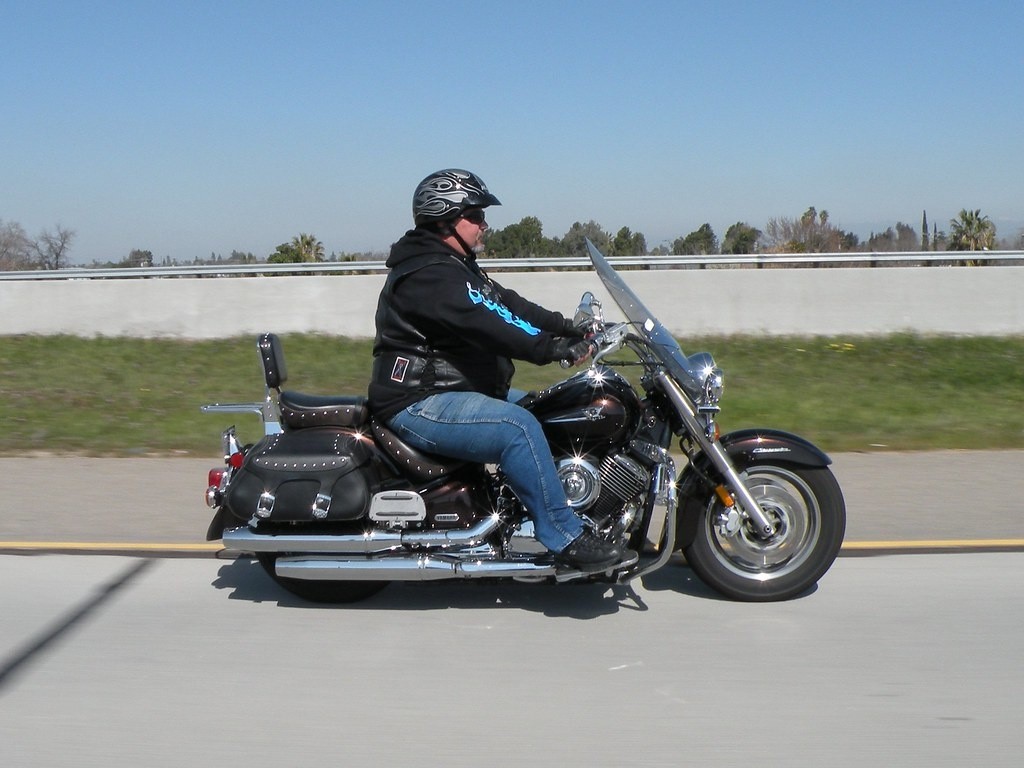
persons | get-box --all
[367,168,640,572]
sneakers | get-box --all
[553,530,622,570]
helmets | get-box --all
[412,168,501,231]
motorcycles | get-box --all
[198,235,848,603]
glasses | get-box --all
[459,209,485,224]
